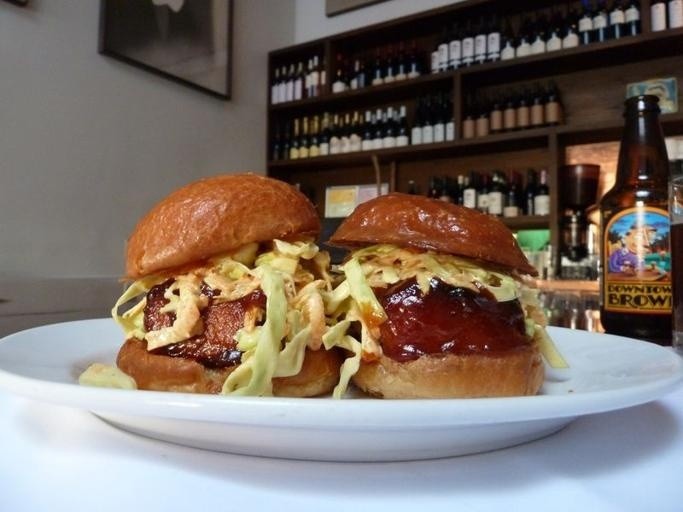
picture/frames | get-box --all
[96,0,233,101]
[325,0,386,17]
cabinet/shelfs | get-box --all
[264,0,682,346]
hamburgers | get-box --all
[323,192,569,398]
[100,171,332,395]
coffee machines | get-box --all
[558,161,604,280]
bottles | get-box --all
[601,94,681,342]
[409,95,457,146]
[503,0,682,76]
[268,55,329,105]
[403,168,550,218]
[272,110,365,160]
[429,20,502,76]
[333,37,424,96]
[462,79,566,142]
[364,105,408,153]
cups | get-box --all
[666,173,683,345]
